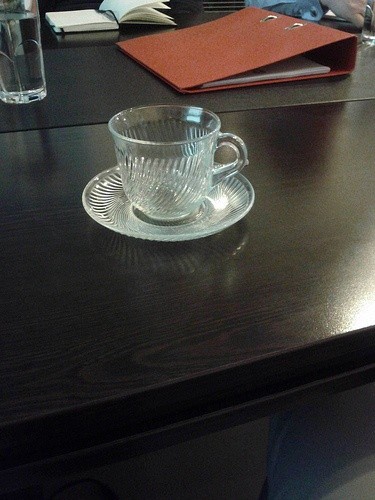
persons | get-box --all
[245,0,375,30]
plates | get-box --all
[81,164,253,241]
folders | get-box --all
[114,6,359,95]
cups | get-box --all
[107,103,248,221]
[0,0,48,105]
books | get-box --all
[44,0,178,34]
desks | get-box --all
[1,13,375,499]
